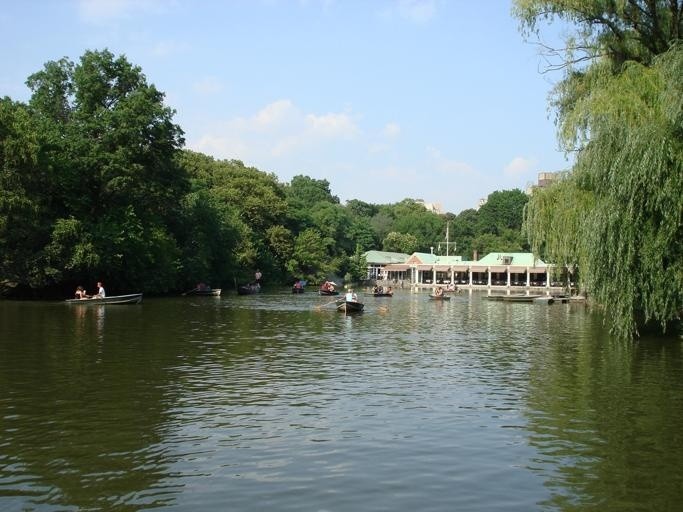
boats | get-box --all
[333,301,365,315]
[483,292,541,303]
[63,293,146,305]
[289,287,306,294]
[178,287,221,297]
[428,293,450,302]
[316,289,340,297]
[238,282,261,294]
[368,291,394,298]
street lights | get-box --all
[437,241,457,255]
[432,256,440,284]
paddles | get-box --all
[181,288,197,295]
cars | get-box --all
[425,278,576,287]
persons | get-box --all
[343,277,457,304]
[196,268,335,293]
[75,282,105,299]
